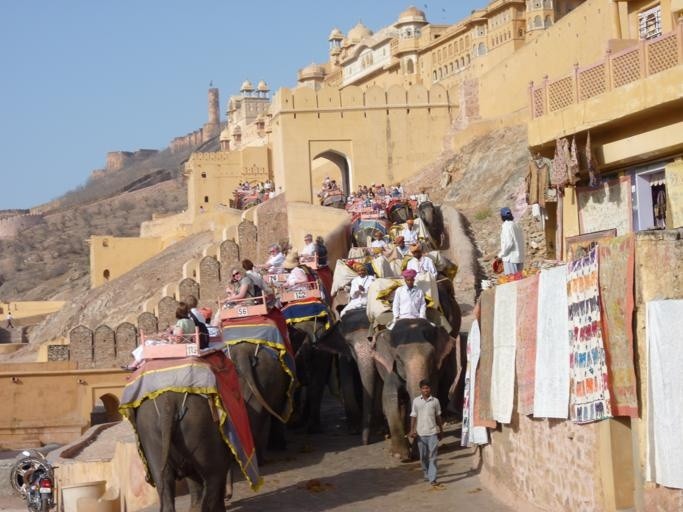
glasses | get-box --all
[233,272,238,277]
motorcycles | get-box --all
[20,462,59,511]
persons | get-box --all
[184,294,205,328]
[5,311,13,328]
[495,204,524,273]
[218,175,448,322]
[199,306,213,324]
[120,302,194,371]
[410,380,444,485]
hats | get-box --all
[407,220,414,224]
[396,235,404,242]
[374,231,383,236]
[282,256,300,269]
[402,269,416,278]
[409,244,421,252]
[500,207,512,217]
[198,306,212,318]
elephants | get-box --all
[322,194,345,209]
[369,317,459,462]
[222,342,289,468]
[313,307,378,447]
[133,390,236,512]
[286,319,332,435]
[388,202,414,223]
[436,274,462,338]
[417,200,446,249]
[355,221,387,246]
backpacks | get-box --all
[189,311,209,349]
[247,277,262,304]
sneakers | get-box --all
[424,477,437,486]
